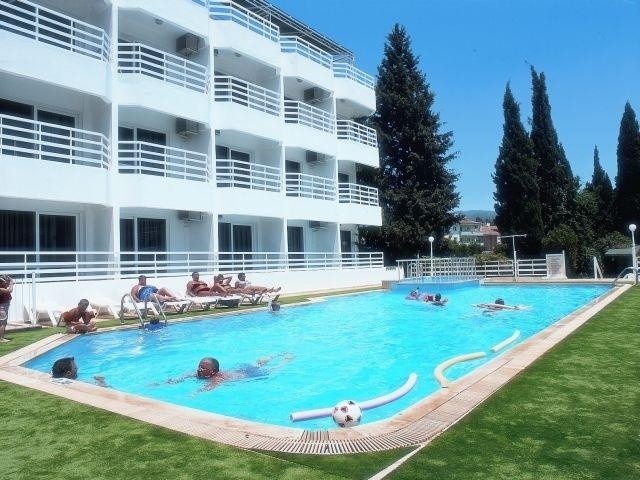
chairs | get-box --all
[24,284,281,328]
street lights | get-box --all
[428,236,435,277]
[629,224,637,280]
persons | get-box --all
[142,318,168,330]
[0,274,14,344]
[470,296,530,317]
[130,276,181,304]
[63,298,97,334]
[404,286,448,307]
[235,273,281,293]
[51,356,107,388]
[213,274,267,297]
[186,272,233,297]
[144,351,294,403]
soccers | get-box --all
[333,400,361,427]
